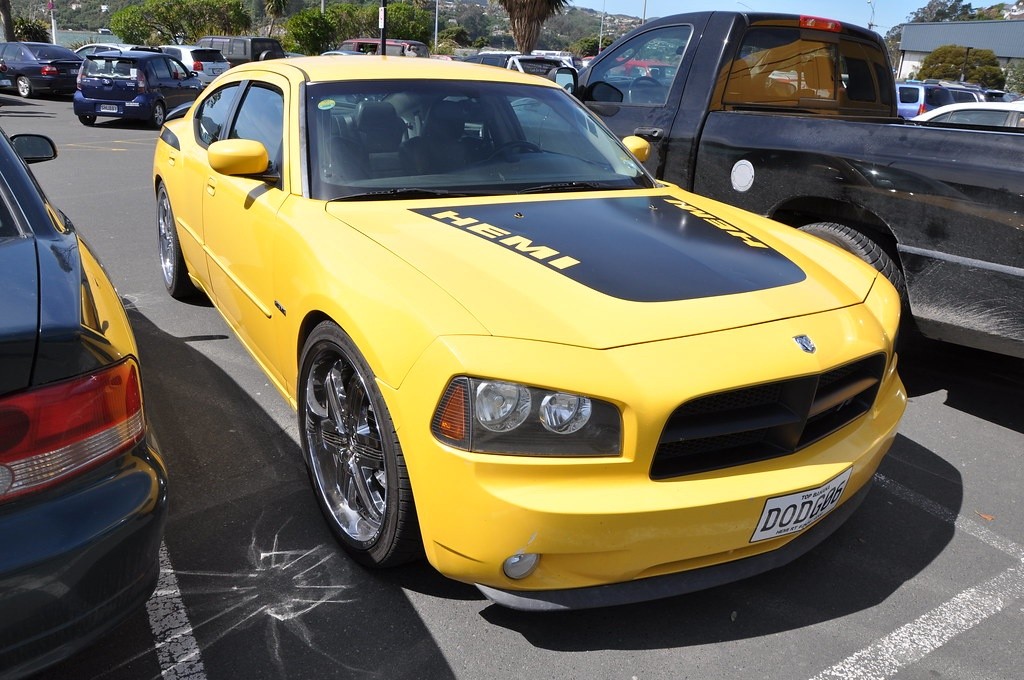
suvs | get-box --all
[192,35,286,66]
[460,54,579,77]
[335,39,430,58]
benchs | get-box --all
[328,101,403,182]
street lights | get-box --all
[867,0,876,31]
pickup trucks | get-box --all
[547,11,1022,360]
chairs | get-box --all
[400,99,478,178]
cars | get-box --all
[478,49,677,85]
[896,76,1024,105]
[157,44,232,87]
[895,81,956,121]
[321,51,364,55]
[152,54,911,615]
[1,42,85,100]
[74,43,163,59]
[430,54,461,62]
[909,100,1024,128]
[72,50,204,128]
[1,128,171,680]
[284,51,306,58]
